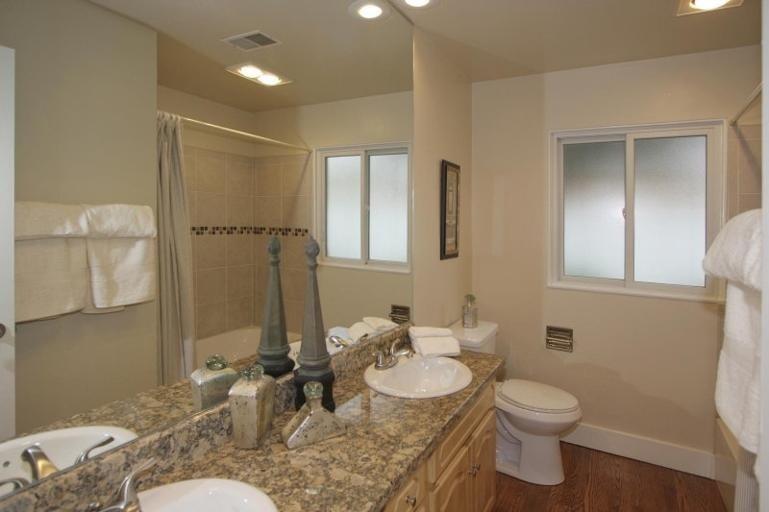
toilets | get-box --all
[449,318,585,487]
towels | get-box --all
[14,201,159,325]
[703,206,765,456]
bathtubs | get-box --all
[195,325,302,368]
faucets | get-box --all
[328,336,350,348]
[20,444,60,482]
[84,499,139,512]
[390,337,414,361]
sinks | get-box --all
[0,425,138,495]
[364,354,474,399]
[287,337,346,370]
[136,478,279,512]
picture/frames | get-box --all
[441,159,459,260]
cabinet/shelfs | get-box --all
[370,378,499,510]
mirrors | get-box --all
[0,1,419,497]
